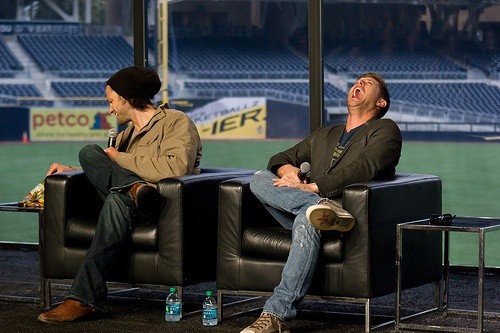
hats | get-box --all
[105,66,161,104]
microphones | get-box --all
[298,161,311,182]
[108,127,118,147]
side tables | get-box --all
[393,215,500,333]
[0,201,43,302]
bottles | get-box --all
[202,291,218,326]
[165,287,181,322]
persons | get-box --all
[38,66,203,325]
[239,74,402,333]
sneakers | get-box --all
[307,200,355,231]
[241,310,287,333]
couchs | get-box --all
[211,173,444,333]
[41,167,259,311]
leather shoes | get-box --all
[132,183,168,214]
[38,299,93,322]
